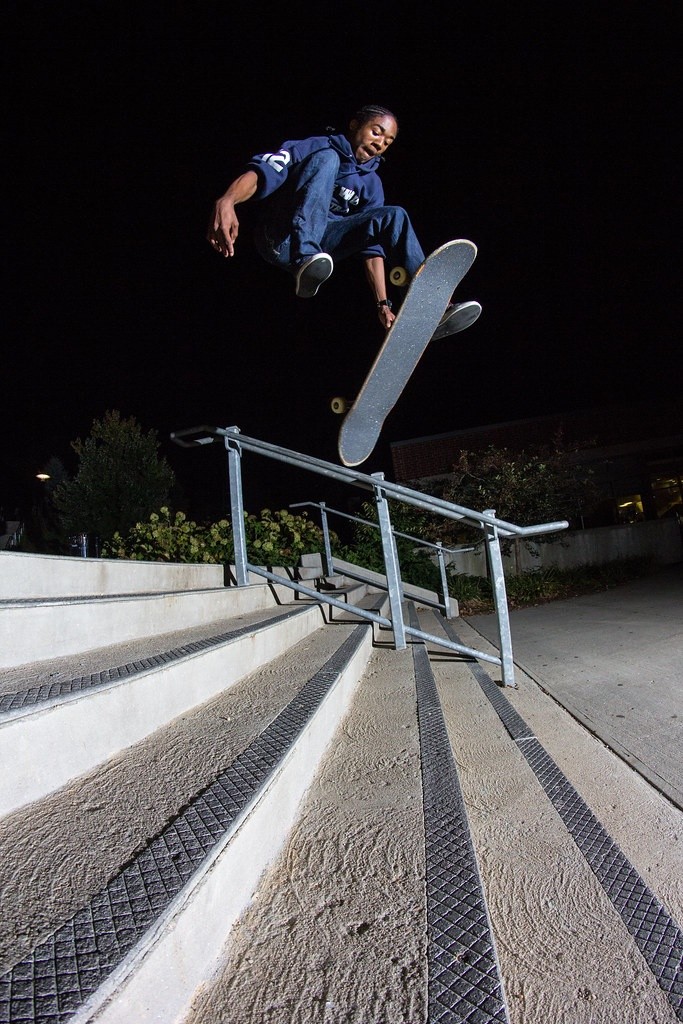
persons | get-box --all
[207,105,482,340]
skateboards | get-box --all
[329,236,479,469]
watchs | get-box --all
[376,299,393,309]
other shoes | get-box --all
[286,252,333,297]
[428,298,483,342]
